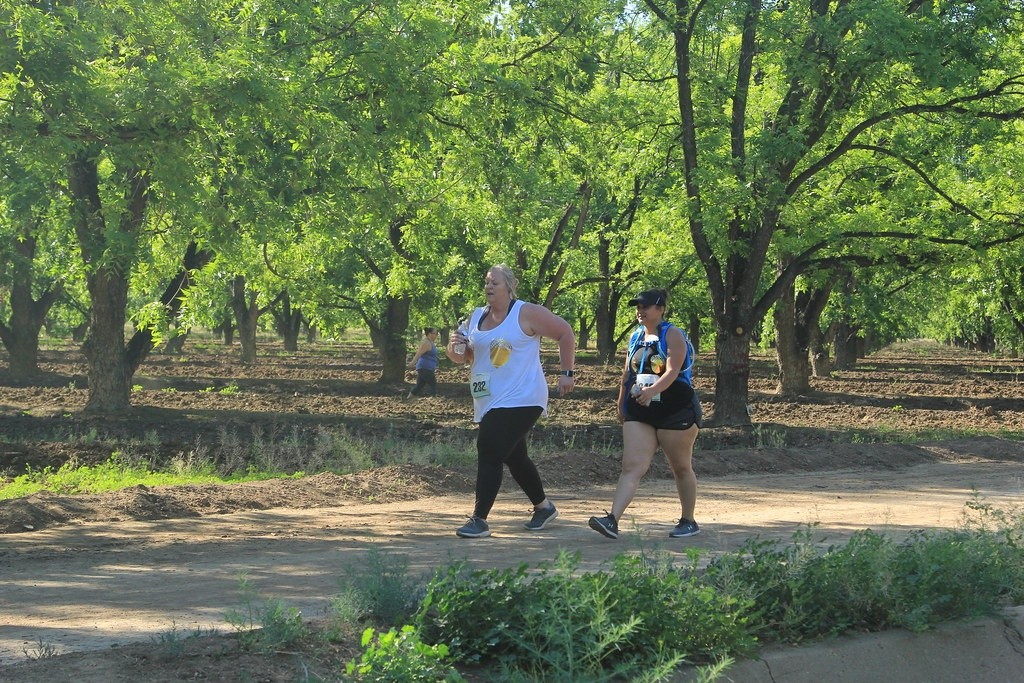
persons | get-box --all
[407,328,440,399]
[588,288,703,539]
[445,262,577,538]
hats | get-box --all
[628,292,666,306]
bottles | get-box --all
[453,321,468,354]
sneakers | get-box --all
[589,508,618,539]
[524,501,558,530]
[456,514,491,538]
[669,518,701,538]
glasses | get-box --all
[430,332,438,335]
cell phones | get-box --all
[630,384,652,407]
[453,332,466,355]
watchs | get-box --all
[562,370,573,377]
